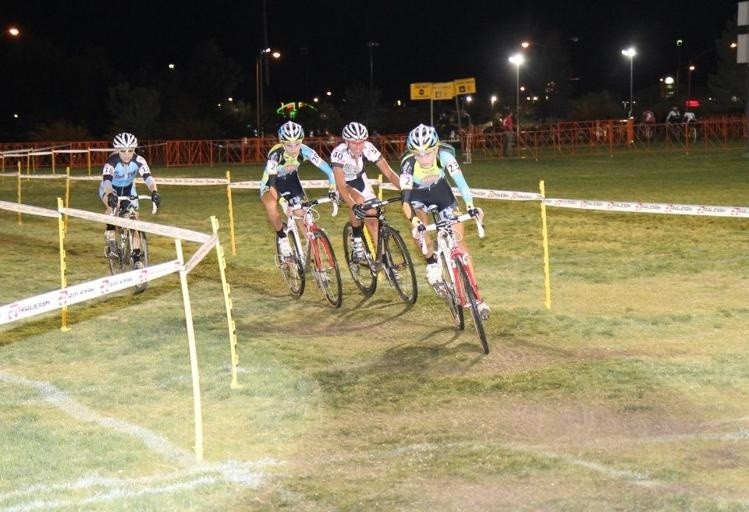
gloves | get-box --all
[283,192,300,207]
[466,206,479,217]
[108,193,118,207]
[353,204,366,220]
[412,216,427,233]
[152,190,160,205]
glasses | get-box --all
[121,150,133,152]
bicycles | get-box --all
[413,204,491,356]
[342,194,418,306]
[274,198,344,310]
[102,194,159,293]
[667,123,698,145]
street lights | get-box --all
[509,54,523,159]
[621,49,635,118]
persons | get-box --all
[97,130,163,271]
[397,123,494,321]
[259,120,338,261]
[328,120,404,258]
[637,105,697,145]
[502,111,515,158]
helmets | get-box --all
[408,124,439,153]
[342,122,368,141]
[113,133,137,148]
[278,121,304,144]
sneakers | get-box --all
[477,303,490,315]
[130,256,144,268]
[278,237,294,257]
[352,240,364,258]
[108,247,119,257]
[427,263,440,285]
